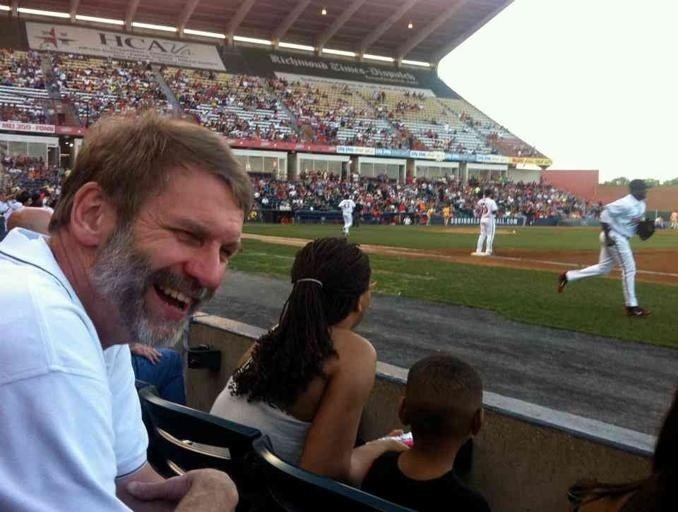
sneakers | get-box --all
[557,269,568,294]
[623,305,654,318]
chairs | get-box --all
[137,382,418,512]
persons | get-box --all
[360,349,494,512]
[472,188,498,257]
[206,234,411,488]
[556,177,655,318]
[654,214,667,229]
[126,335,191,408]
[668,208,677,232]
[1,108,260,512]
[1,155,72,236]
[562,383,676,512]
[244,168,604,227]
[2,46,540,158]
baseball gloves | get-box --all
[637,218,654,240]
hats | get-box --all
[627,179,654,191]
